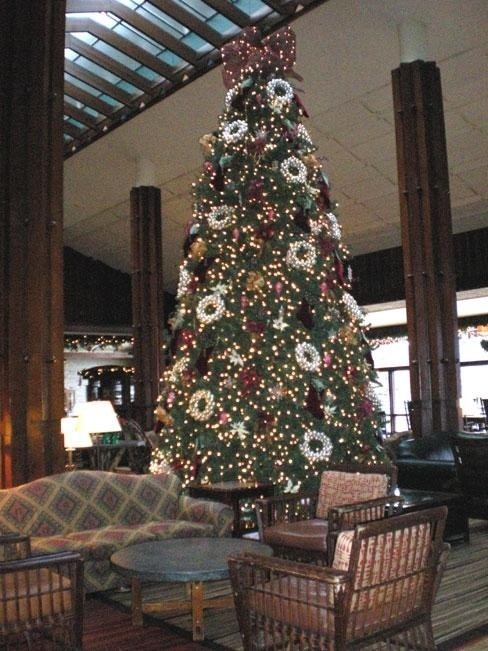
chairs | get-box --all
[227,502,453,649]
[263,463,398,566]
[449,431,488,520]
[0,531,84,651]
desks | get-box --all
[79,440,146,473]
[187,479,273,539]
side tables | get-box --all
[385,488,470,548]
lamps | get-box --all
[59,400,123,469]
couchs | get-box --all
[0,469,236,597]
[384,430,488,493]
